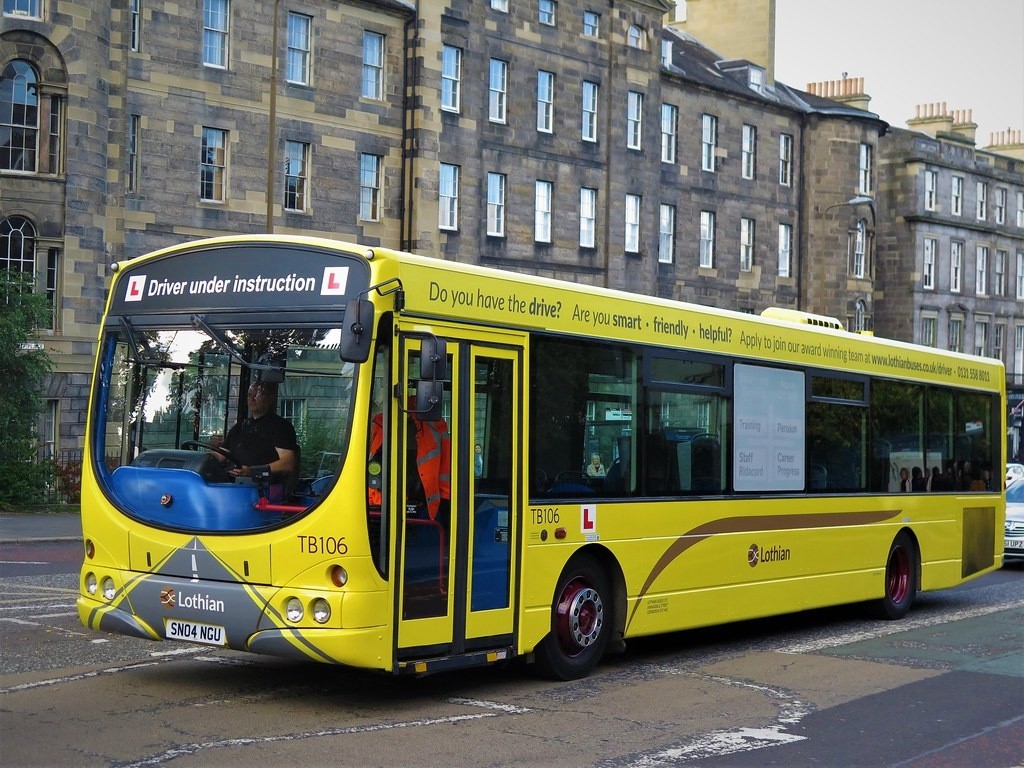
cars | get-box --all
[1003,476,1024,566]
[1004,463,1024,489]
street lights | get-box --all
[819,196,874,316]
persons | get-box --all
[205,379,300,503]
[899,454,1024,492]
[474,444,483,479]
[368,395,451,520]
[586,454,606,476]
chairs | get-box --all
[473,423,992,500]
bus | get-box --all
[77,233,1003,683]
